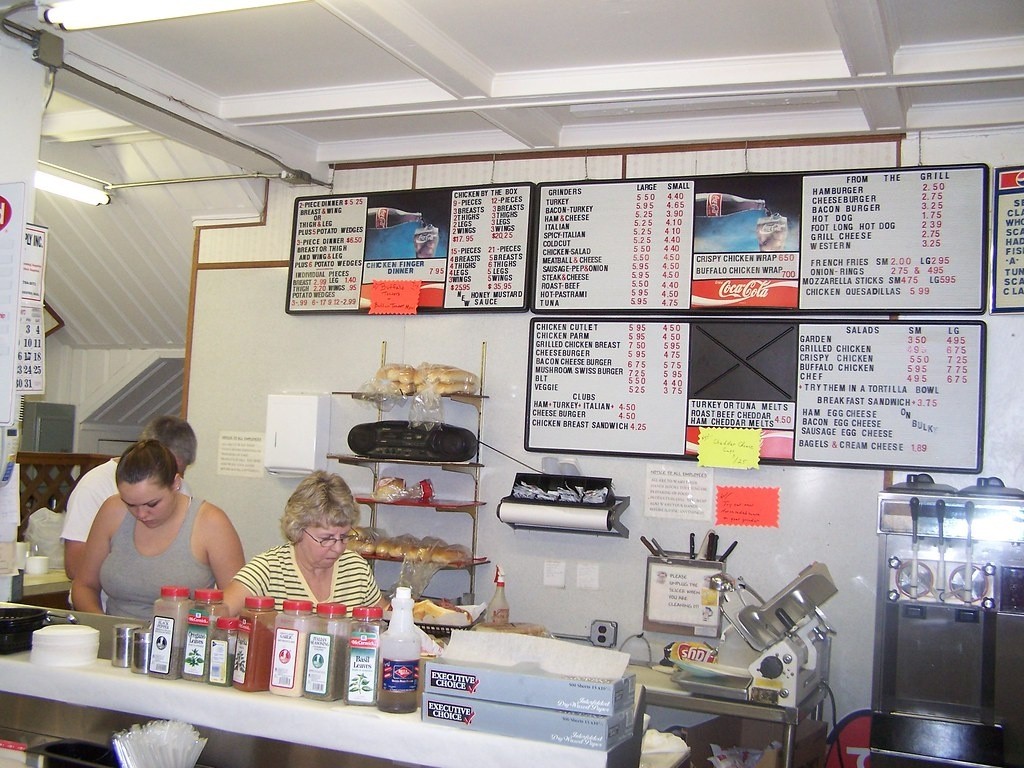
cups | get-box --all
[414,228,439,258]
[26,556,49,574]
[757,217,787,251]
[17,543,30,568]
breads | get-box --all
[373,477,406,500]
[411,598,453,621]
[384,366,478,396]
[345,527,466,563]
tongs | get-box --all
[45,610,79,624]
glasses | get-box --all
[302,526,359,547]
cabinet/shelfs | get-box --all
[325,337,488,642]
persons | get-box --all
[224,470,390,619]
[64,415,246,620]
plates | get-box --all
[31,624,100,667]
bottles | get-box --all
[378,587,419,714]
[366,207,423,229]
[696,193,765,217]
[181,589,228,683]
[131,631,152,674]
[112,624,144,667]
[303,603,350,701]
[269,600,314,697]
[486,564,510,624]
[148,586,193,680]
[344,607,387,705]
[209,618,238,687]
[232,596,277,692]
